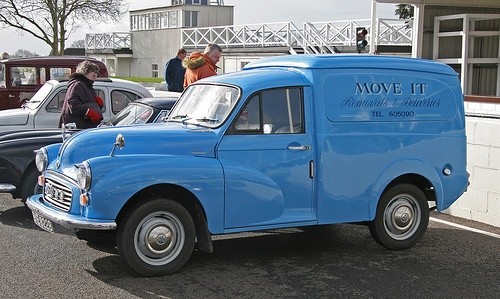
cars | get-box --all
[0,98,179,206]
[0,81,155,133]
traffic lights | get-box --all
[355,27,369,51]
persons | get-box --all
[181,43,222,89]
[164,48,186,93]
[58,60,104,129]
[1,52,21,86]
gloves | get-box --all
[84,108,101,124]
[94,95,103,109]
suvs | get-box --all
[25,53,471,277]
[0,56,111,111]
